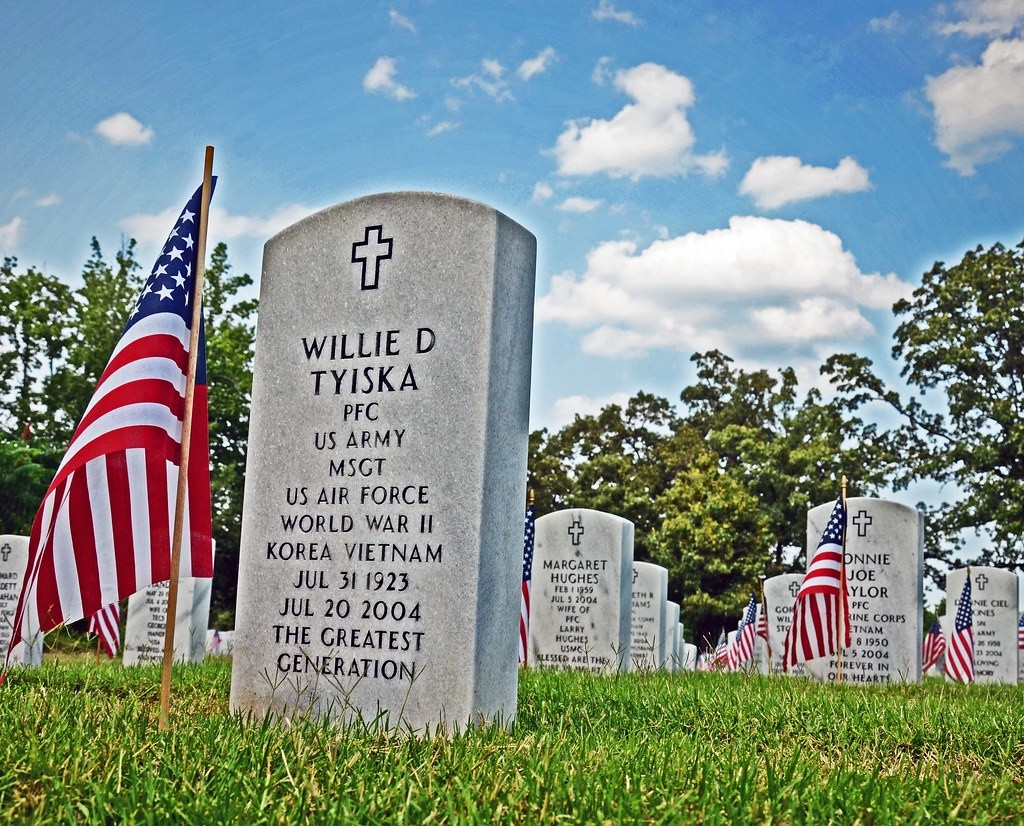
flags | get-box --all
[0,175,219,685]
[756,596,769,642]
[943,576,975,686]
[783,494,851,672]
[1018,612,1024,649]
[696,632,729,672]
[729,595,757,668]
[517,505,535,664]
[922,618,946,672]
